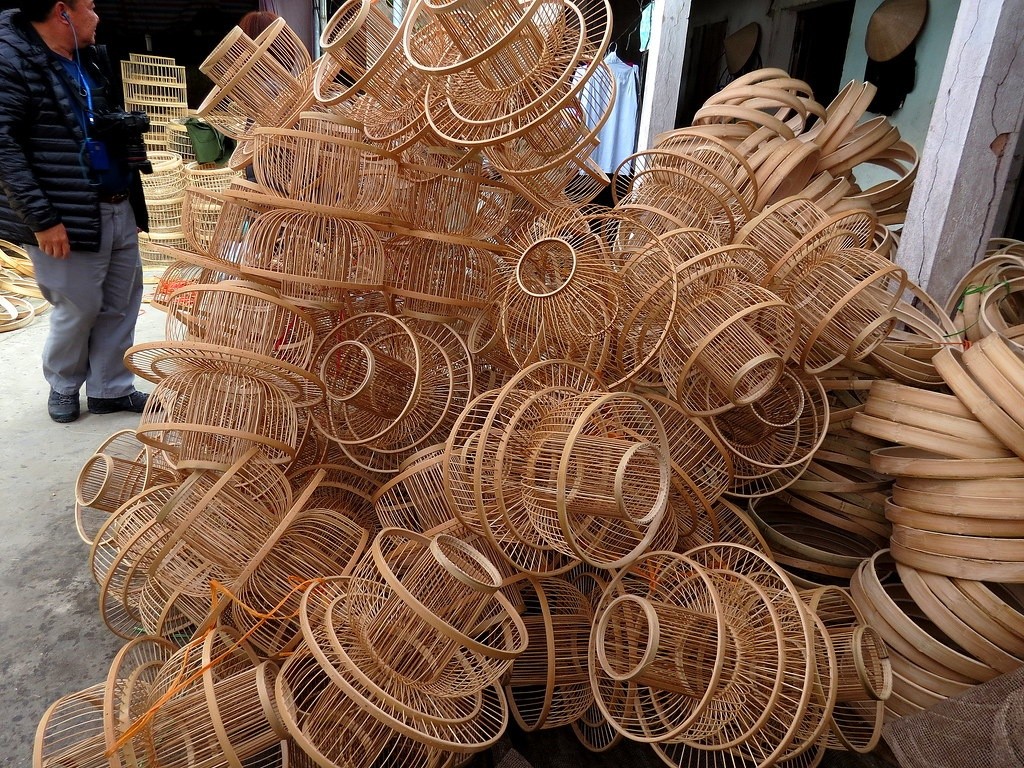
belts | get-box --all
[98,189,131,204]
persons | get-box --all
[1,0,163,423]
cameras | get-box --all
[97,106,154,174]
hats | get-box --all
[723,22,760,76]
[865,0,927,62]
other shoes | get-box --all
[47,386,80,424]
[88,391,168,414]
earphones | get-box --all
[61,11,69,19]
